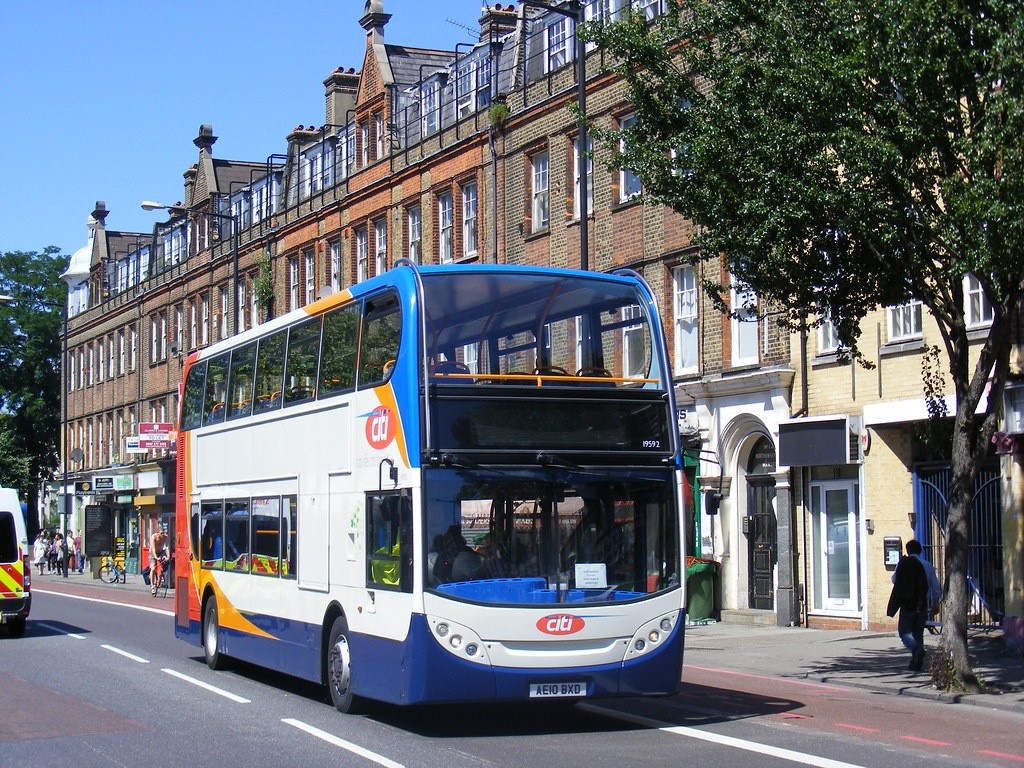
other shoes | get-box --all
[908,660,922,671]
[150,587,155,594]
[909,648,925,669]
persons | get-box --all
[148,527,171,594]
[891,540,942,671]
[73,529,84,574]
[66,531,76,571]
[34,531,47,576]
[56,534,67,575]
[48,531,57,574]
[433,505,636,591]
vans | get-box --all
[0,489,32,637]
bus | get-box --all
[174,258,723,713]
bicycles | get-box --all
[151,557,171,599]
[99,551,126,584]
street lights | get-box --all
[0,295,68,579]
[142,201,238,335]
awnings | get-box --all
[862,386,995,429]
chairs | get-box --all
[256,395,271,410]
[271,391,281,407]
[474,377,503,385]
[532,366,575,387]
[211,402,225,420]
[577,368,617,388]
[430,361,475,384]
[312,380,344,398]
[383,360,396,379]
[504,372,533,385]
[232,403,243,416]
[239,399,251,415]
[291,386,312,400]
